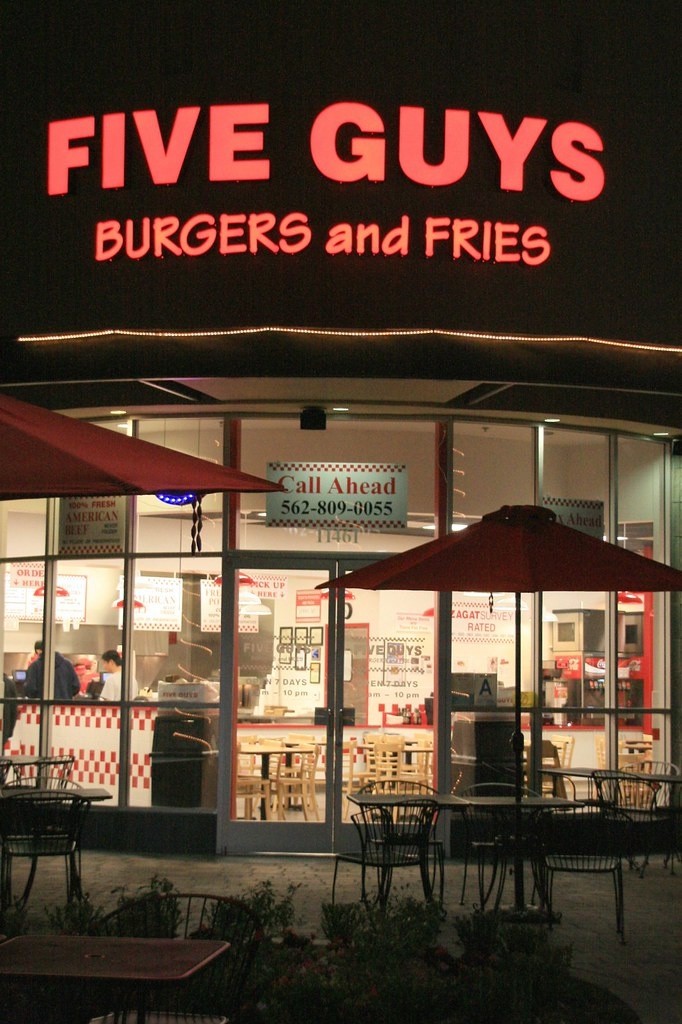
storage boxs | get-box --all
[156,681,220,717]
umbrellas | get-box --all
[0,394,288,501]
[314,503,681,907]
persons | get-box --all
[97,649,139,701]
[23,641,81,701]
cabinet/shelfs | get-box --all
[542,608,643,726]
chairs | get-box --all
[237,732,325,821]
[594,733,656,810]
[343,733,434,824]
[459,759,682,942]
[87,893,264,1024]
[523,735,576,812]
[331,779,444,913]
[0,756,92,902]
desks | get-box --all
[0,934,231,1024]
[625,737,653,771]
[537,768,682,870]
[239,742,314,820]
[356,744,434,790]
[0,755,75,788]
[0,788,114,912]
[346,794,585,923]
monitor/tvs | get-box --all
[426,698,472,725]
[314,708,355,727]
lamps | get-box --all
[421,608,435,617]
[239,592,272,616]
[113,599,144,609]
[33,585,70,598]
[321,589,355,603]
[299,405,327,430]
[337,600,338,603]
[213,572,255,587]
